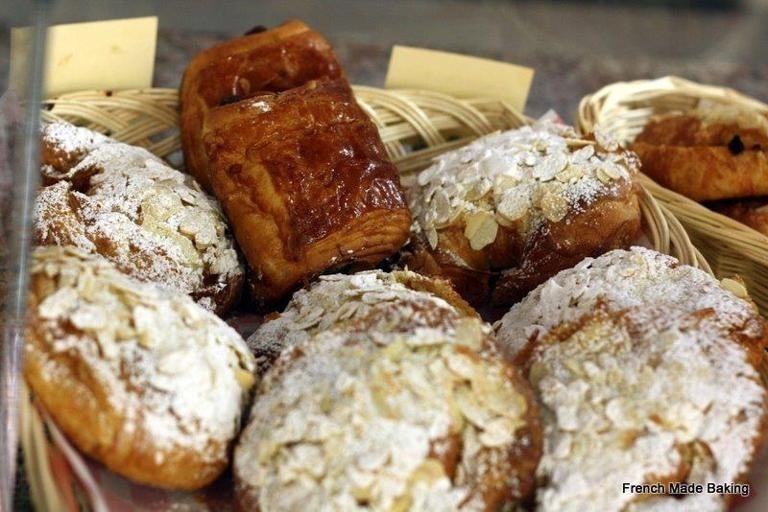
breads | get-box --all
[20,18,768,512]
